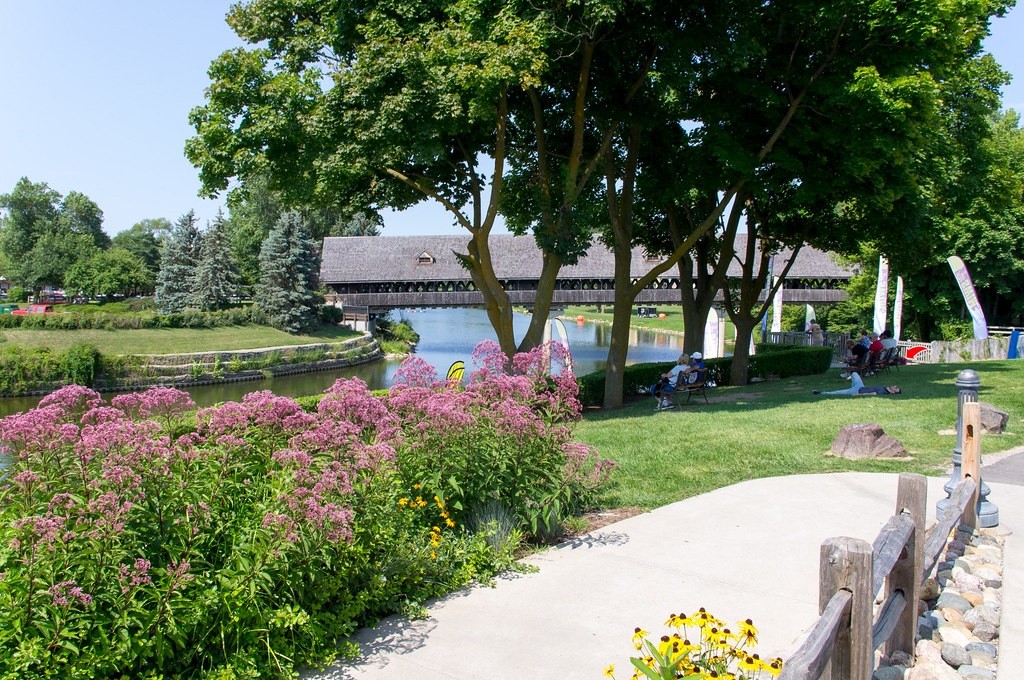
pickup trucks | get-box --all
[11,304,57,315]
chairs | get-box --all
[846,345,902,379]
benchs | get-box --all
[654,368,709,411]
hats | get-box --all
[690,352,702,359]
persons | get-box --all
[839,329,898,378]
[649,351,705,409]
[811,372,901,396]
[802,318,824,346]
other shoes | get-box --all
[870,372,874,375]
[663,405,674,408]
[864,373,870,376]
[841,373,850,378]
[653,406,665,411]
[812,390,821,395]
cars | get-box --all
[43,290,125,304]
[0,303,20,316]
